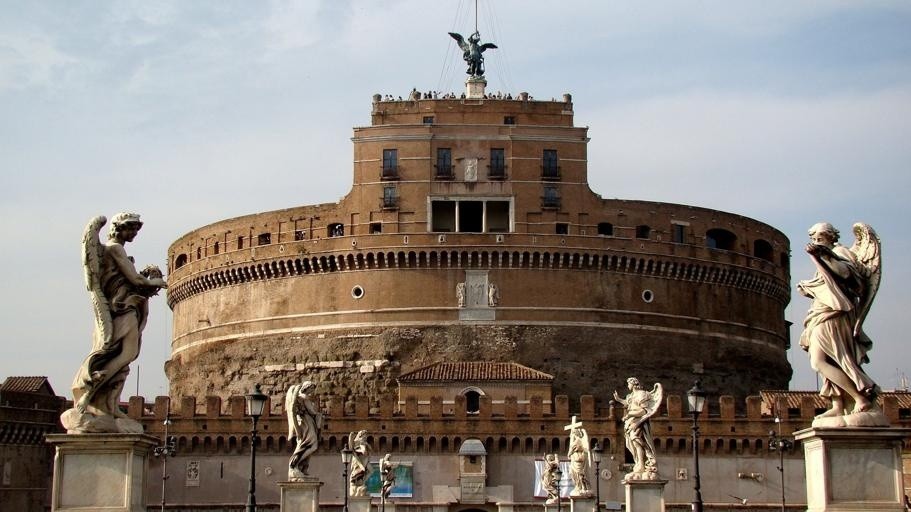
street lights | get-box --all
[241,382,266,512]
[378,469,387,512]
[685,379,707,512]
[152,420,176,512]
[338,444,352,512]
[773,408,785,512]
[590,444,601,512]
[554,466,562,512]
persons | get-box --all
[467,30,485,76]
[283,381,324,485]
[565,427,594,496]
[379,454,394,503]
[349,428,373,496]
[540,452,562,505]
[798,217,894,434]
[56,210,169,433]
[383,86,534,102]
[611,377,664,484]
[456,283,466,306]
[488,283,499,304]
